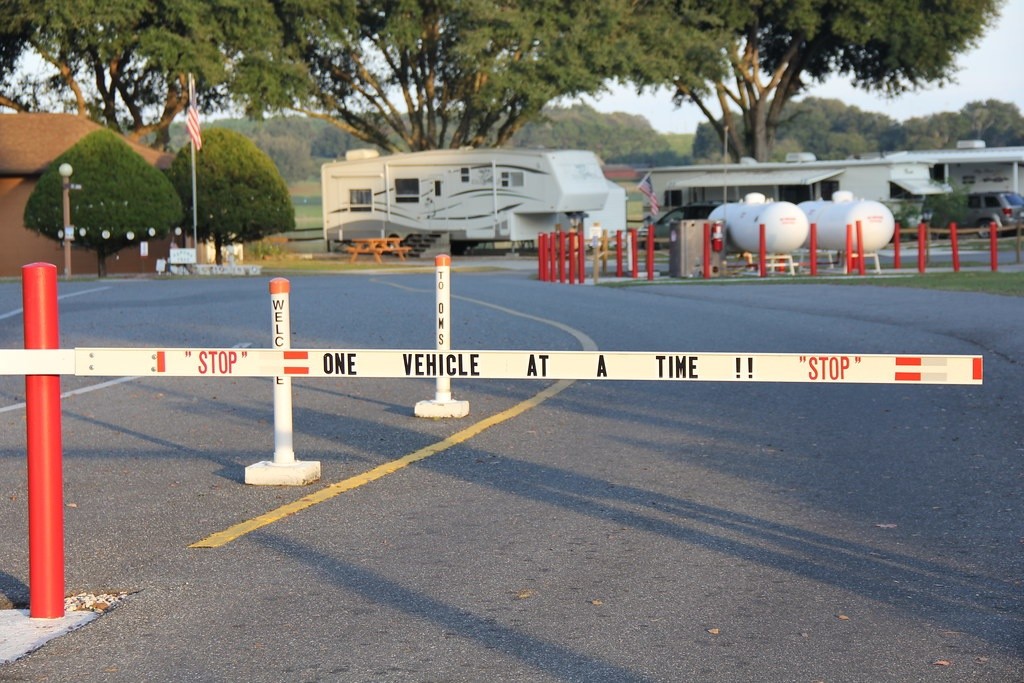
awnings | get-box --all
[681,169,844,202]
[890,179,952,195]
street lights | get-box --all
[59,164,73,280]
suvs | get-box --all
[638,200,726,252]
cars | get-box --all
[930,189,1023,240]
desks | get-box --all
[351,238,405,264]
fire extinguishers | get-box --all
[712,220,724,251]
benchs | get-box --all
[345,247,411,253]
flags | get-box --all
[186,93,203,151]
[639,176,658,215]
